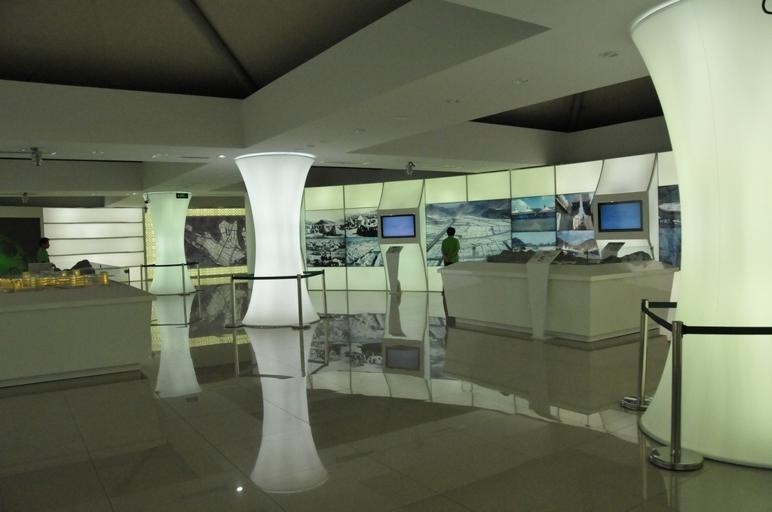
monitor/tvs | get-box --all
[597,199,643,232]
[381,214,416,239]
[386,346,419,370]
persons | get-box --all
[439,226,461,296]
[36,237,50,262]
